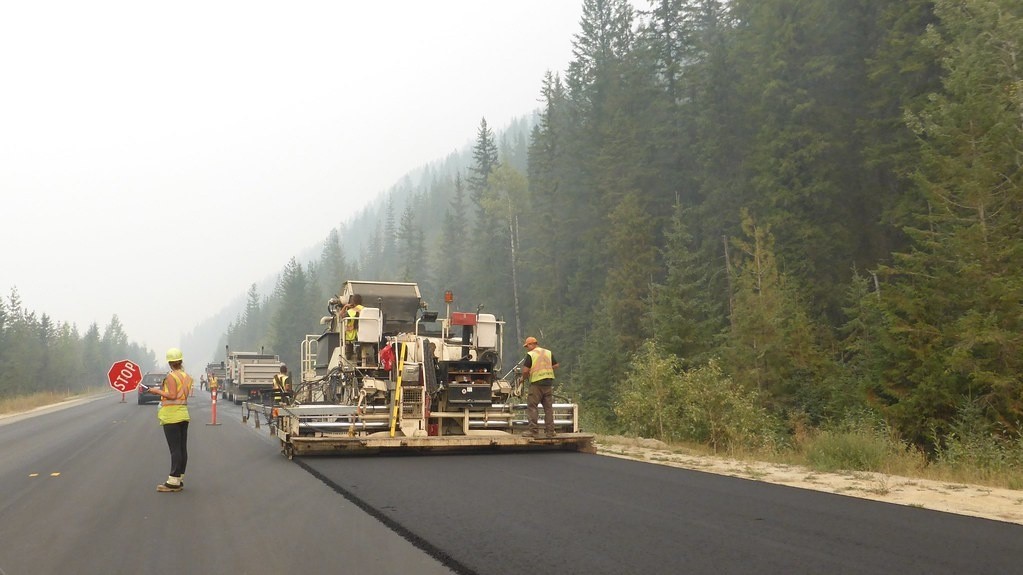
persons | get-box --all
[272,366,298,406]
[200,375,207,391]
[210,373,219,402]
[518,337,560,437]
[339,294,366,340]
[146,348,193,492]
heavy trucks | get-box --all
[204,345,284,403]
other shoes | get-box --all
[546,430,556,437]
[523,432,539,437]
[157,482,184,492]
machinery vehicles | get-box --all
[273,280,600,459]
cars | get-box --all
[137,370,168,406]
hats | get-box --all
[523,336,537,347]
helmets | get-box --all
[166,349,183,361]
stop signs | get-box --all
[107,359,142,393]
